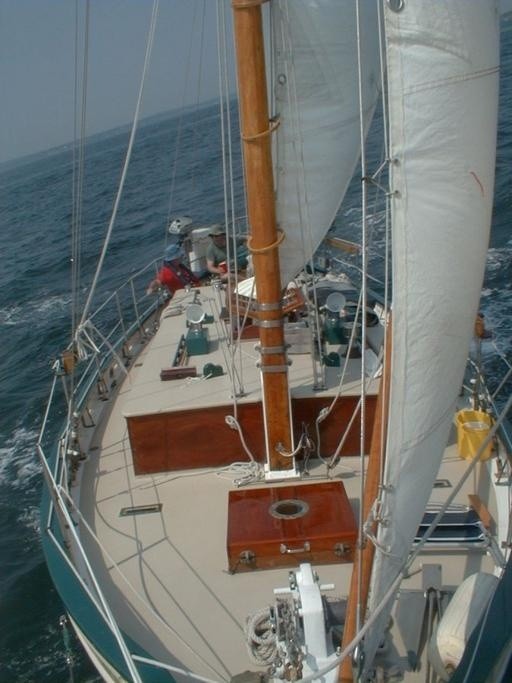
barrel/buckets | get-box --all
[456,410,496,463]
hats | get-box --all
[207,224,226,236]
[229,256,249,273]
[162,244,184,261]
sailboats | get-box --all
[36,0,512,681]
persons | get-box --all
[146,244,201,297]
[205,223,248,275]
